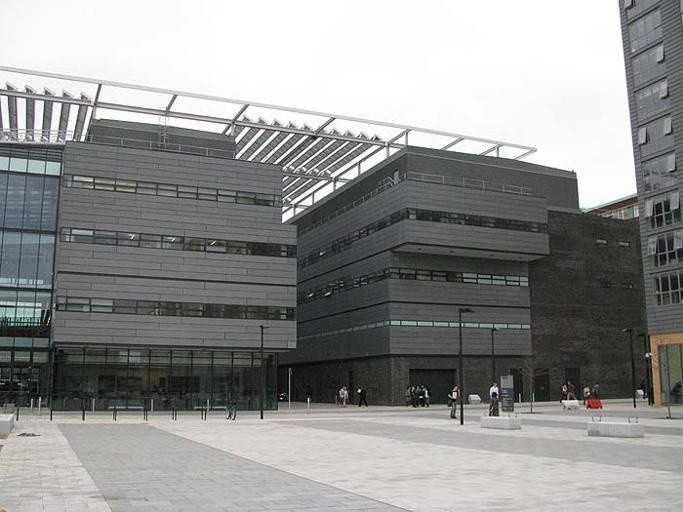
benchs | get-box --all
[191,399,228,410]
[0,413,15,435]
[561,400,579,409]
[107,399,144,410]
[587,414,643,437]
[636,389,646,399]
[479,411,520,429]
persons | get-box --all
[559,380,601,406]
[336,381,501,419]
[640,378,647,399]
[225,395,239,420]
[304,384,313,403]
[671,381,682,401]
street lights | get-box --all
[457,308,475,425]
[258,324,269,418]
[490,325,499,387]
[638,334,652,408]
[622,327,638,409]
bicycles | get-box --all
[225,400,236,419]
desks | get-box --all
[672,393,682,403]
